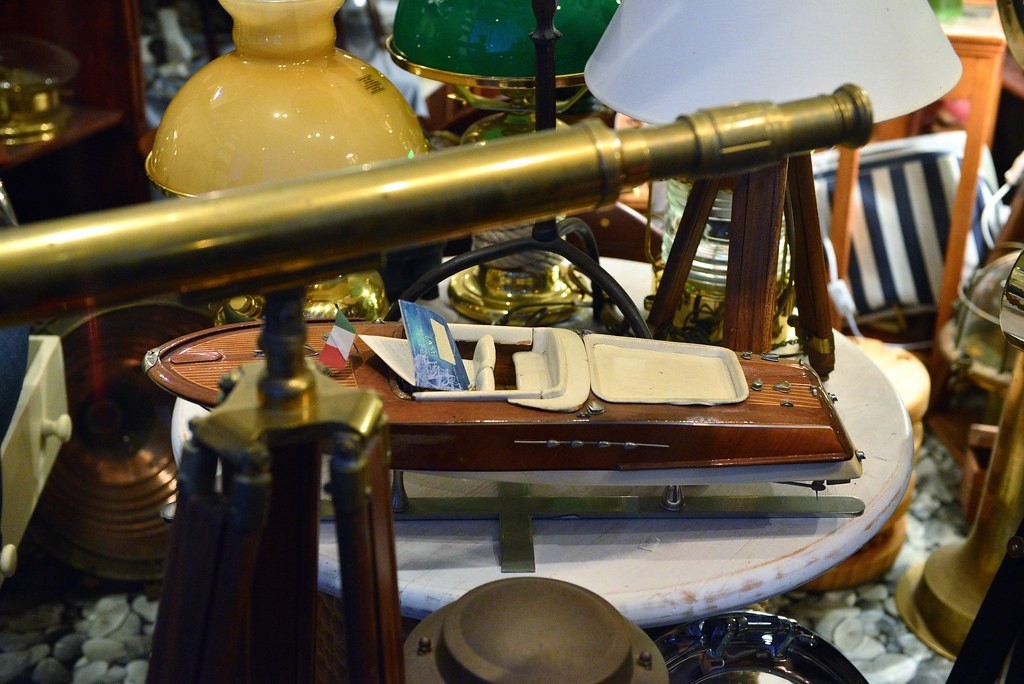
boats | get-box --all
[141,310,866,484]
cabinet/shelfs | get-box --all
[1,1,147,232]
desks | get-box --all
[169,254,914,684]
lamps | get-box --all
[583,0,964,352]
[386,0,621,323]
